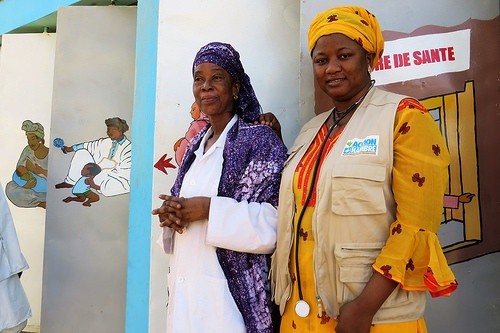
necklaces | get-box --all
[329,110,348,130]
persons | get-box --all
[152,41,287,333]
[0,185,31,333]
[252,4,459,333]
